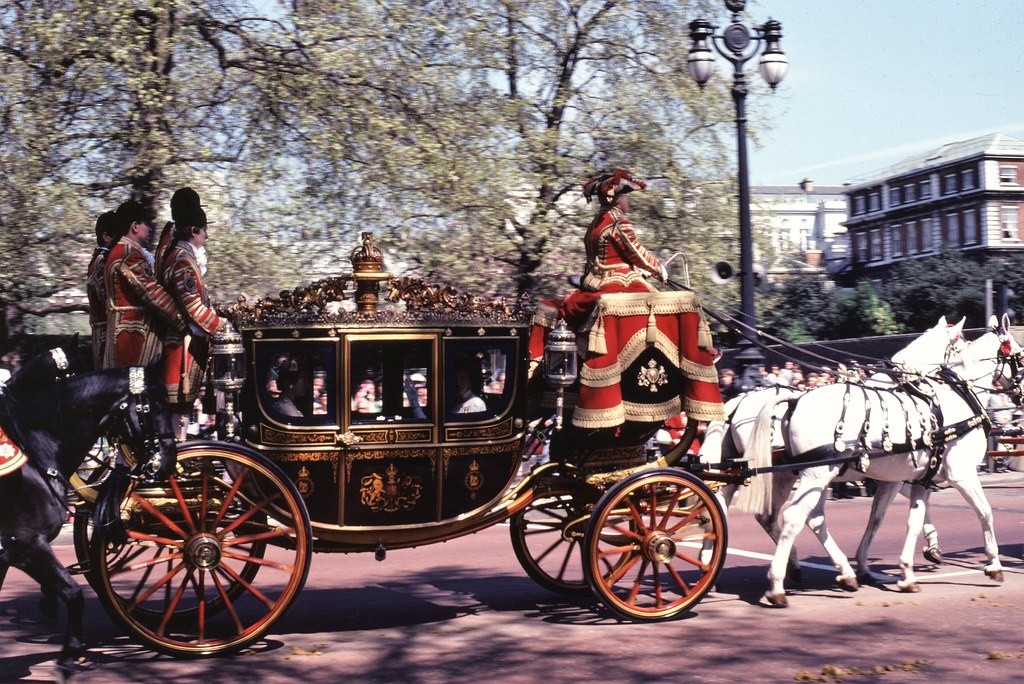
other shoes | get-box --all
[712,348,723,363]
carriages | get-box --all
[73,230,1020,659]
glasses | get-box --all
[619,193,630,197]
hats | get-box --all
[96,211,118,235]
[175,205,215,228]
[170,187,208,221]
[582,167,646,205]
[456,364,480,383]
[116,201,156,231]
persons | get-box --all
[656,361,1024,500]
[582,171,722,363]
[178,352,554,499]
[87,188,226,445]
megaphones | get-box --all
[751,263,765,288]
[711,259,735,285]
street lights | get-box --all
[685,0,789,383]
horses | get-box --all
[728,312,1024,609]
[698,315,966,592]
[0,332,178,674]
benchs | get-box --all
[563,292,704,347]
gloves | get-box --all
[659,264,668,284]
